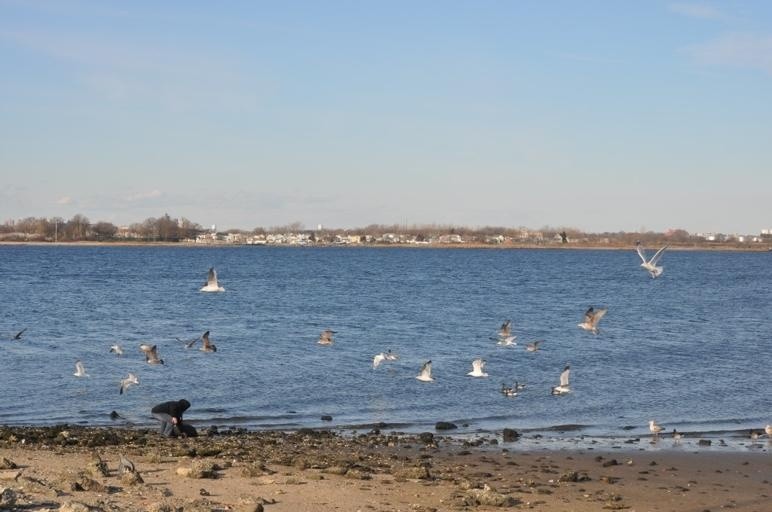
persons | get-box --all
[152,399,190,440]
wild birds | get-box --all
[749,426,767,443]
[109,344,123,356]
[175,330,218,353]
[648,419,666,440]
[139,343,164,364]
[635,238,669,280]
[8,327,31,342]
[577,304,609,337]
[198,265,226,294]
[414,358,436,384]
[527,339,545,352]
[764,424,772,437]
[496,319,519,347]
[72,359,91,380]
[465,356,490,378]
[315,328,339,347]
[671,427,686,446]
[120,371,140,395]
[500,380,526,398]
[551,365,573,397]
[371,348,397,372]
